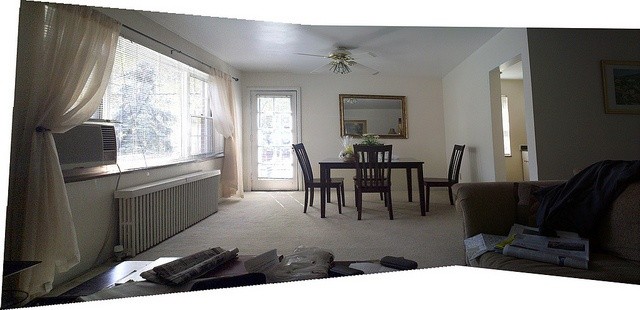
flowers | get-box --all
[361,132,379,145]
[341,143,357,154]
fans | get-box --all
[295,51,380,76]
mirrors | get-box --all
[339,94,408,139]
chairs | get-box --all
[293,143,345,214]
[353,145,393,220]
[352,151,386,206]
[423,145,465,212]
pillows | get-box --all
[512,182,558,222]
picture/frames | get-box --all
[344,120,367,135]
[601,59,640,114]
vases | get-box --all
[367,151,376,163]
[342,156,356,163]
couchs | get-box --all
[451,159,639,284]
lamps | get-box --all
[327,47,355,75]
[345,98,359,105]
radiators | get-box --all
[114,170,220,257]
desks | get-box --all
[318,158,425,218]
[56,257,406,301]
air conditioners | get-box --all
[57,122,117,166]
